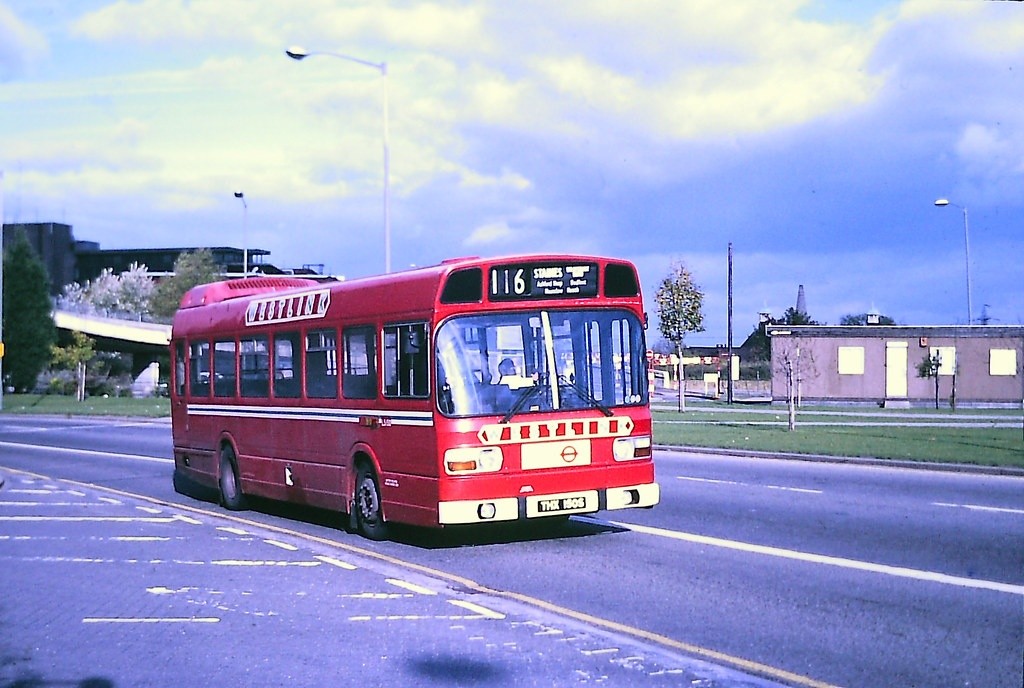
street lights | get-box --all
[286,42,392,275]
[934,199,973,325]
[233,191,250,277]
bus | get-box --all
[168,252,659,541]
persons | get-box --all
[496,358,518,412]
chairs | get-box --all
[193,362,557,415]
